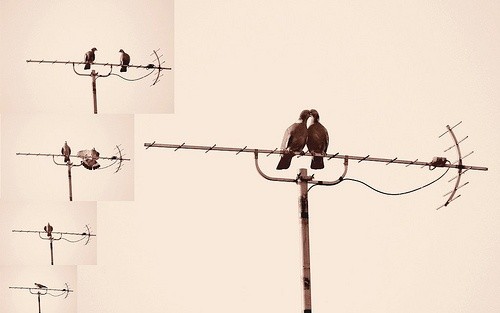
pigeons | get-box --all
[34,283,48,289]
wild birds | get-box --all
[276,109,313,170]
[117,48,130,73]
[61,141,71,163]
[44,222,54,237]
[84,47,98,70]
[76,148,101,170]
[308,109,330,169]
